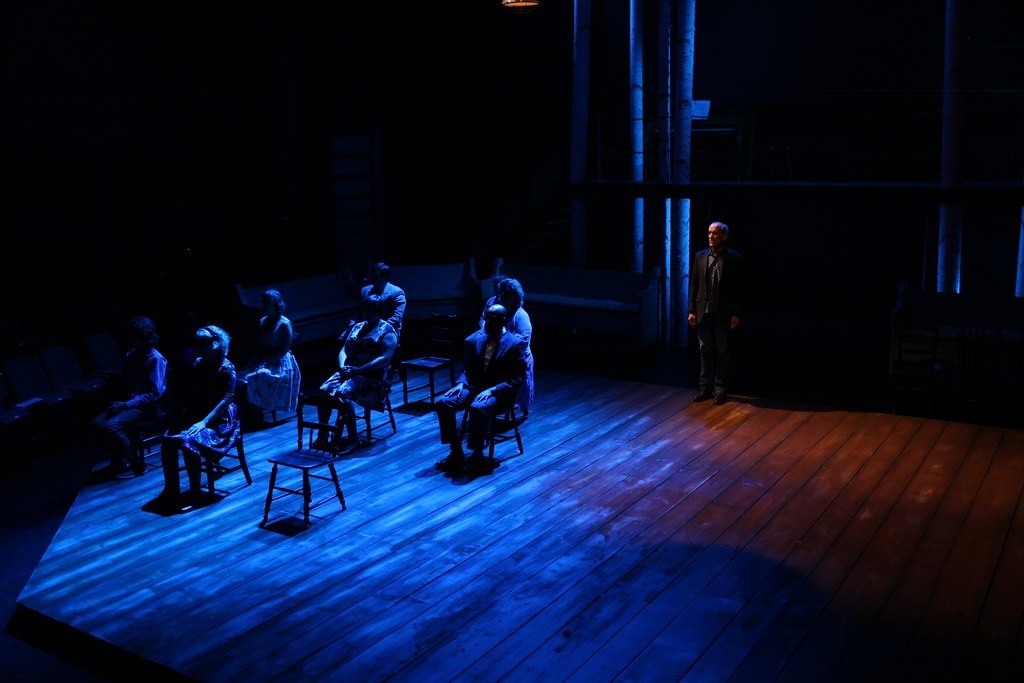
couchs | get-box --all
[375,264,474,319]
[242,273,373,347]
[505,264,659,340]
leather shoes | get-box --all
[714,393,728,404]
[692,389,716,401]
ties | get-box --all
[484,340,494,370]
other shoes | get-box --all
[311,434,329,449]
[436,449,465,468]
[337,437,360,456]
[460,451,486,471]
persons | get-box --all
[496,278,534,416]
[478,274,509,329]
[348,263,406,340]
[311,294,399,455]
[152,324,240,508]
[687,222,744,405]
[236,288,302,429]
[88,315,168,479]
[436,303,525,474]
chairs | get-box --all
[459,392,523,460]
[401,313,457,409]
[887,325,1024,417]
[260,393,345,529]
[178,436,253,505]
[332,345,396,449]
[1,333,123,423]
[126,418,166,475]
